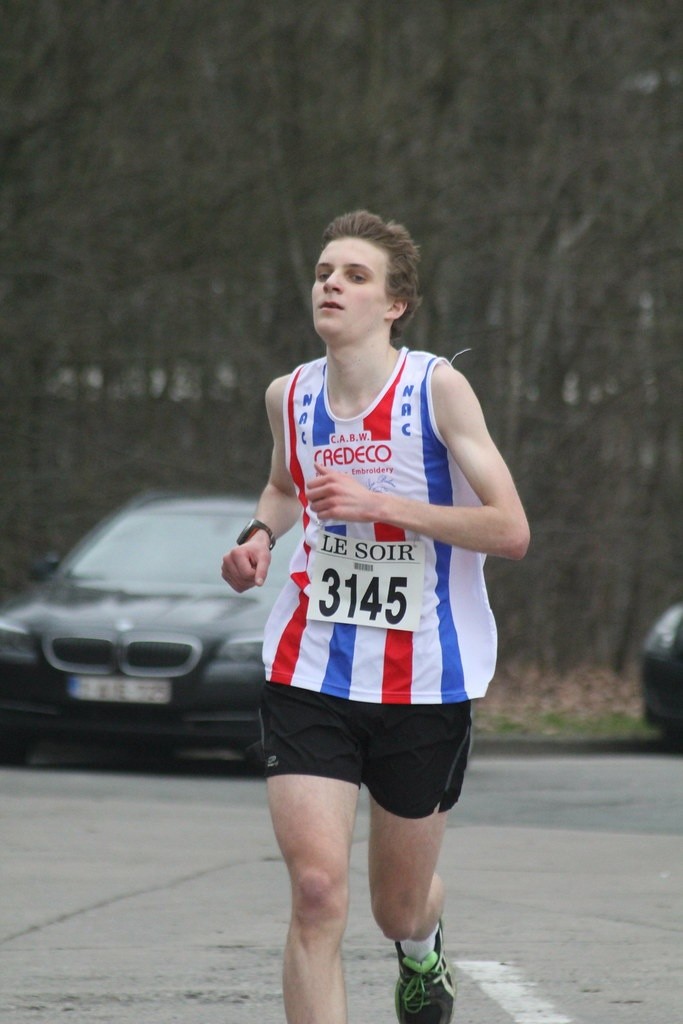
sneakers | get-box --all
[395,919,455,1024]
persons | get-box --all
[219,209,532,1024]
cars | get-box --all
[0,487,307,776]
[637,598,683,755]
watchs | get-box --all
[236,518,276,550]
